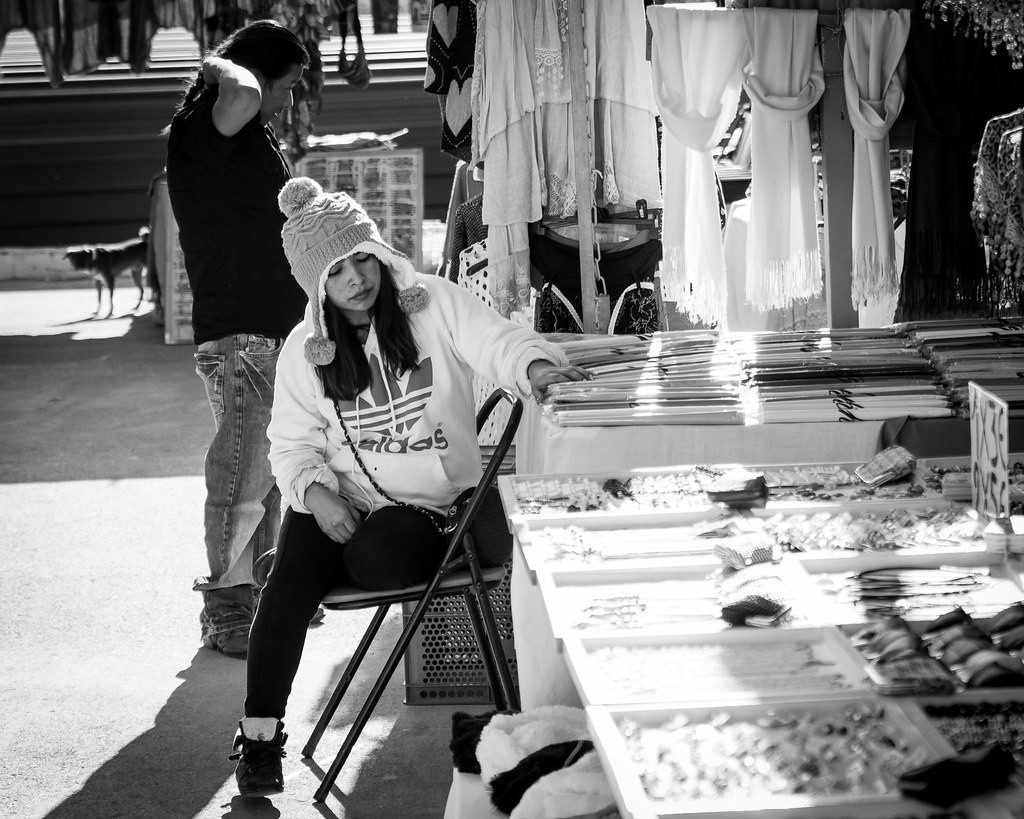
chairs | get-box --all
[303,387,523,802]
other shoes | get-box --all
[252,584,327,625]
[252,547,276,587]
[228,717,288,798]
[200,585,255,656]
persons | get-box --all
[229,178,593,794]
[166,20,325,655]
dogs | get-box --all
[61,226,150,320]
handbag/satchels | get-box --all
[445,484,512,572]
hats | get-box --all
[277,175,429,365]
